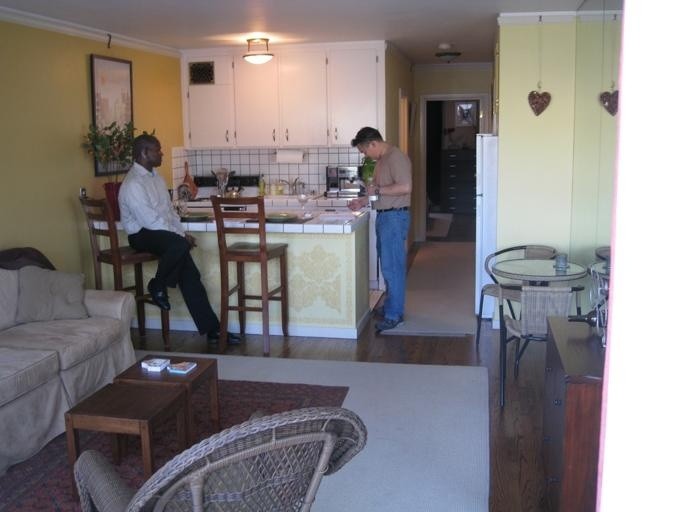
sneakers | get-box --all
[374,304,405,331]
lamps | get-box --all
[243,39,274,64]
[435,46,460,64]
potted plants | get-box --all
[81,121,137,222]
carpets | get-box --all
[0,379,350,512]
[426,212,453,237]
[133,349,490,512]
[380,247,477,337]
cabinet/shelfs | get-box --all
[327,48,378,148]
[188,56,236,150]
[441,149,477,213]
[542,316,605,512]
[279,51,328,148]
[234,54,281,149]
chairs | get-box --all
[210,195,289,357]
[476,245,555,348]
[74,407,367,512]
[79,198,169,336]
[499,283,585,407]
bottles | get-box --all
[368,177,379,201]
[182,161,198,200]
[258,174,266,196]
[276,179,284,195]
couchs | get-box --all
[0,247,137,479]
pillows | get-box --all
[17,266,89,323]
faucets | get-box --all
[279,176,300,195]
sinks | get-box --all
[263,194,322,200]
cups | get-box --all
[366,176,378,201]
[554,254,569,271]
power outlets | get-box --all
[80,188,87,196]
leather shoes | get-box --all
[206,330,240,344]
[149,279,171,310]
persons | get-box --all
[347,127,412,332]
[117,135,242,344]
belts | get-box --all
[375,206,409,213]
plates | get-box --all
[265,213,297,222]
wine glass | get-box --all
[297,190,309,218]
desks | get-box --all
[490,259,588,282]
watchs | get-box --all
[375,184,379,194]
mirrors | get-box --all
[420,92,489,248]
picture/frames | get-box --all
[454,102,477,126]
[90,53,134,176]
